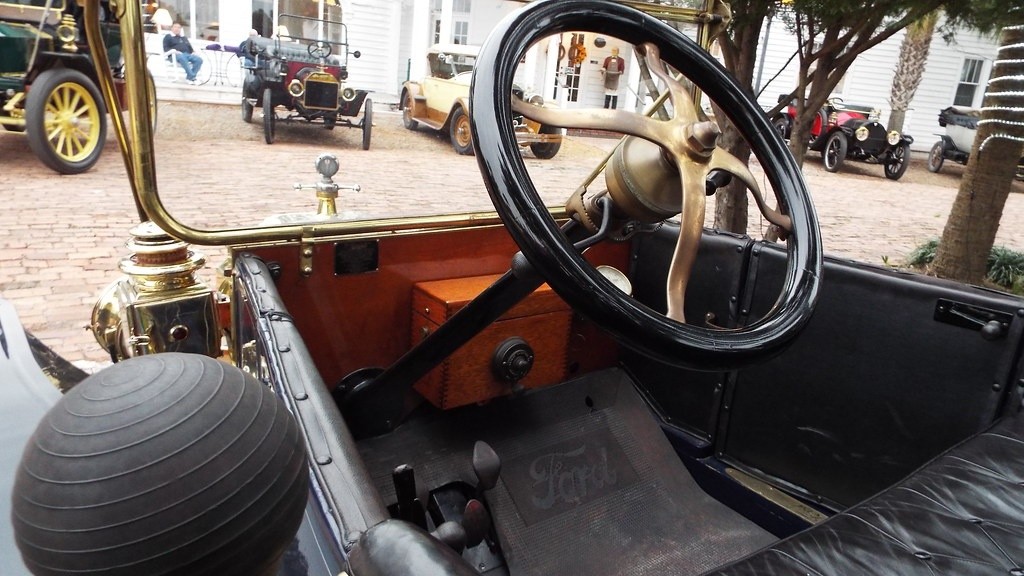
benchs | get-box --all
[684,403,1024,576]
[948,114,978,130]
[251,37,340,64]
[439,64,473,79]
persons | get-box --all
[602,46,625,109]
[163,21,202,85]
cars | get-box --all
[0,0,156,176]
[0,1,1024,575]
[929,103,1024,182]
[243,14,373,150]
[398,42,564,159]
[771,94,914,180]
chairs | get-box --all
[164,48,202,85]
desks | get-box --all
[200,48,239,88]
